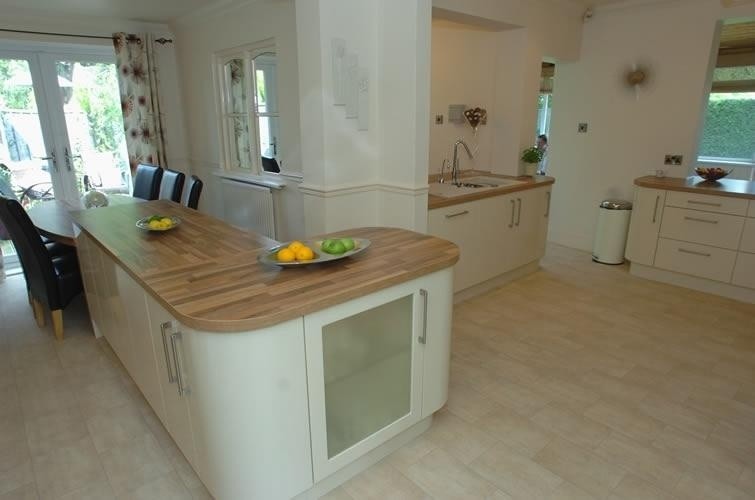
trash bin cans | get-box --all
[592,199,633,265]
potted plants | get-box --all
[521,147,543,175]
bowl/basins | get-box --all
[692,167,734,182]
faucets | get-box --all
[451,140,474,181]
[439,158,450,183]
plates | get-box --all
[257,236,371,268]
[135,214,181,232]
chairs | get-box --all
[159,168,185,204]
[180,175,203,209]
[133,165,162,201]
[2,198,86,339]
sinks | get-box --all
[456,174,529,188]
[428,182,488,198]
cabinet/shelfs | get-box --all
[538,175,555,268]
[625,176,667,279]
[145,290,192,478]
[304,272,429,488]
[653,177,748,298]
[427,192,480,294]
[480,186,537,284]
[731,194,754,303]
